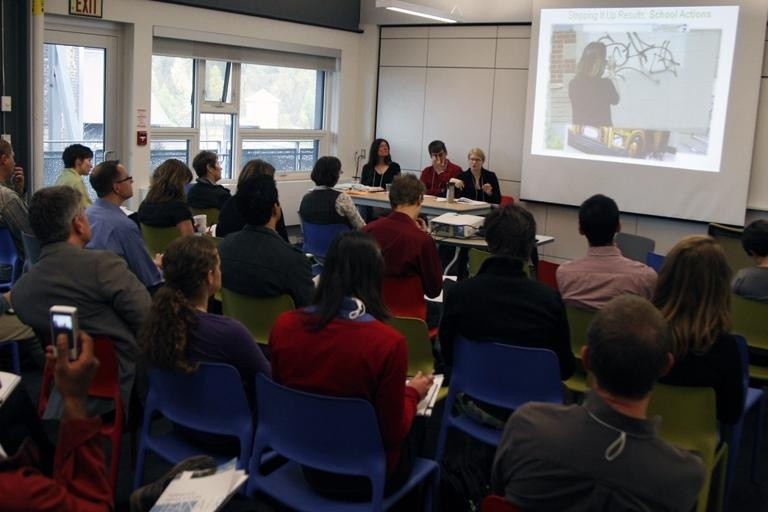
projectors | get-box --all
[429,211,486,240]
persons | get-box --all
[567,41,621,128]
[0,137,766,510]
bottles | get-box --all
[446,181,456,203]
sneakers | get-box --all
[127,453,218,511]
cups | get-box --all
[385,183,393,194]
[193,214,206,234]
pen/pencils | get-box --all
[208,221,214,231]
[422,369,436,376]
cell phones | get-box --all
[49,303,79,362]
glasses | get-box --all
[113,175,133,184]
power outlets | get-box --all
[361,149,365,158]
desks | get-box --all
[335,183,494,219]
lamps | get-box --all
[376,0,463,24]
